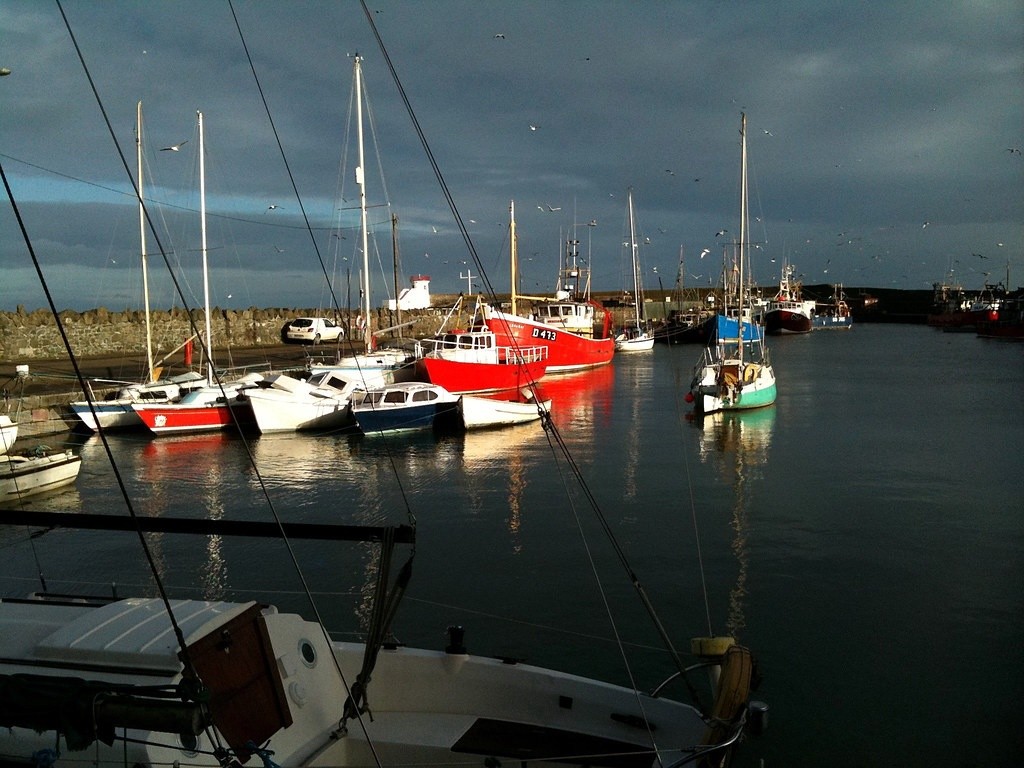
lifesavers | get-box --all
[449,330,465,334]
[742,364,758,383]
[724,371,737,385]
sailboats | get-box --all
[689,108,779,416]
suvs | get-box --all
[285,316,345,346]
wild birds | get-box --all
[622,226,1003,290]
[376,10,383,14]
[532,252,537,256]
[424,253,429,258]
[665,170,677,177]
[580,257,586,264]
[433,226,440,234]
[922,222,930,228]
[274,246,286,254]
[536,203,562,213]
[529,124,539,131]
[264,205,285,215]
[159,141,189,152]
[762,128,773,136]
[469,220,475,224]
[332,235,348,241]
[493,32,505,39]
[756,216,761,222]
[1004,147,1022,156]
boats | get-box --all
[352,380,466,436]
[0,0,755,768]
[0,414,20,456]
[70,48,857,438]
[0,444,84,504]
[455,392,552,432]
[926,251,1024,342]
